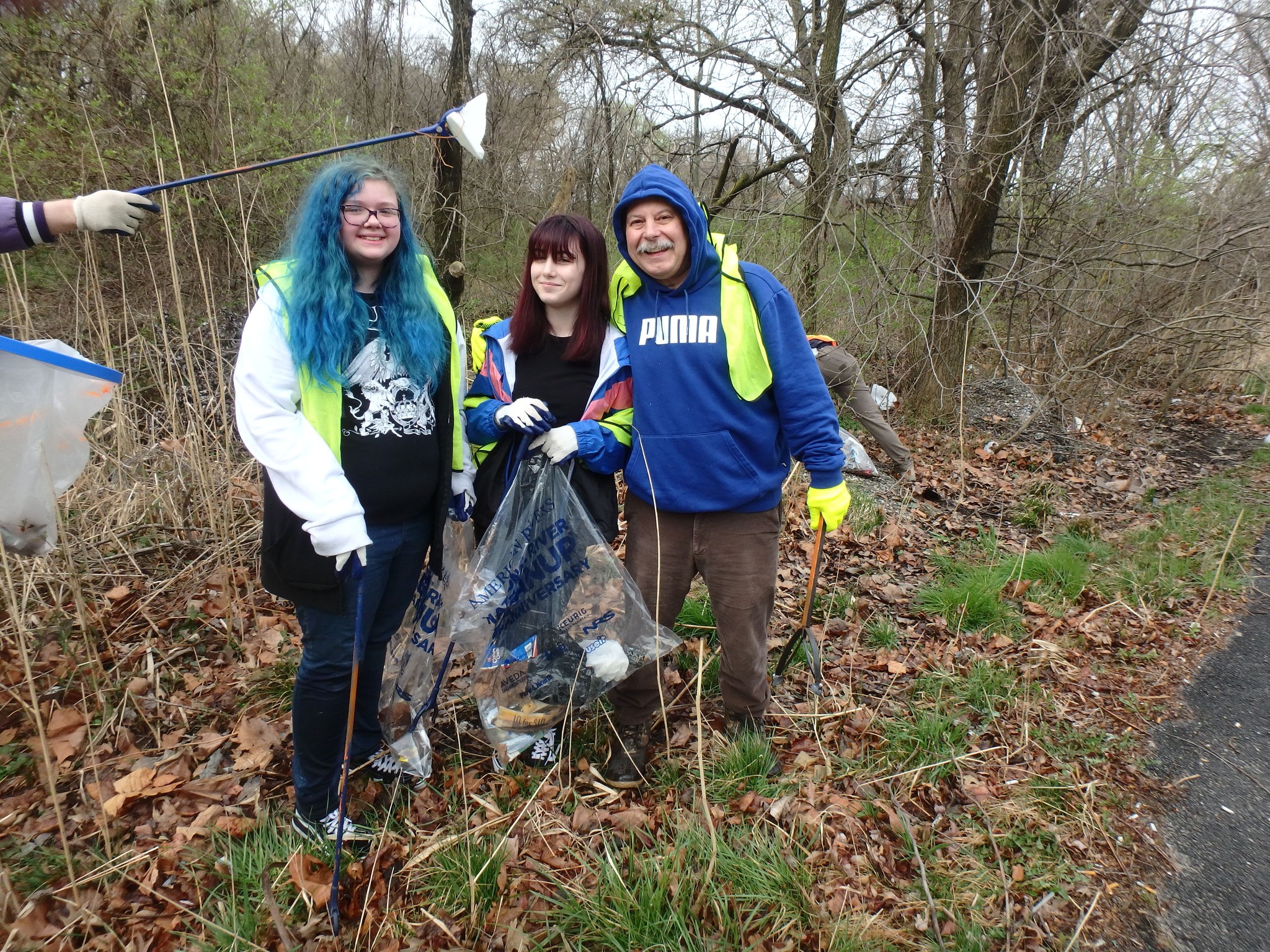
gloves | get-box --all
[448,492,474,523]
[494,398,557,440]
[806,480,853,532]
[528,424,577,466]
[326,547,368,571]
[73,190,160,237]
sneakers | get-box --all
[341,750,425,789]
[493,748,508,774]
[606,714,652,787]
[522,725,565,769]
[292,807,371,859]
[722,707,781,779]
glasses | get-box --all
[340,203,404,228]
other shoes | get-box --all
[897,463,916,481]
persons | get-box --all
[0,190,160,253]
[465,213,632,776]
[805,335,917,481]
[471,167,851,788]
[234,158,476,855]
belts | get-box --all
[816,342,832,350]
